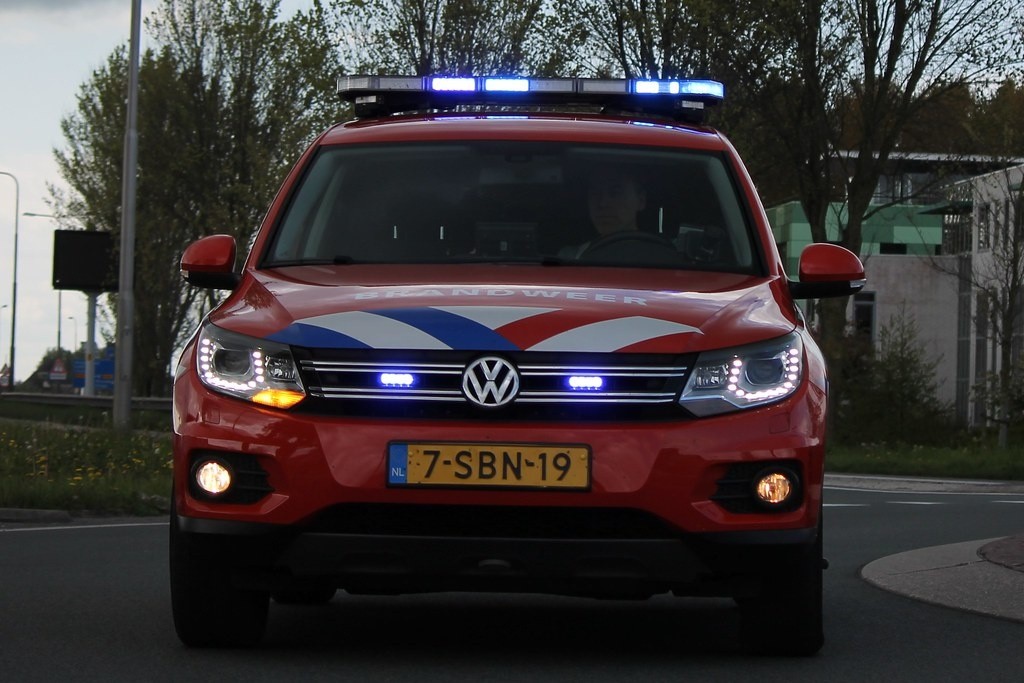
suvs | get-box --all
[168,109,869,657]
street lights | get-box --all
[21,213,63,358]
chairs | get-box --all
[362,183,446,256]
